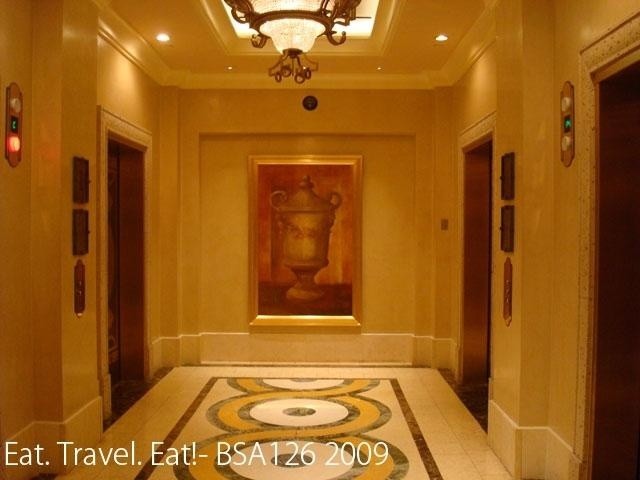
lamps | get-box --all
[224,0,361,83]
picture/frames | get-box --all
[246,155,364,328]
